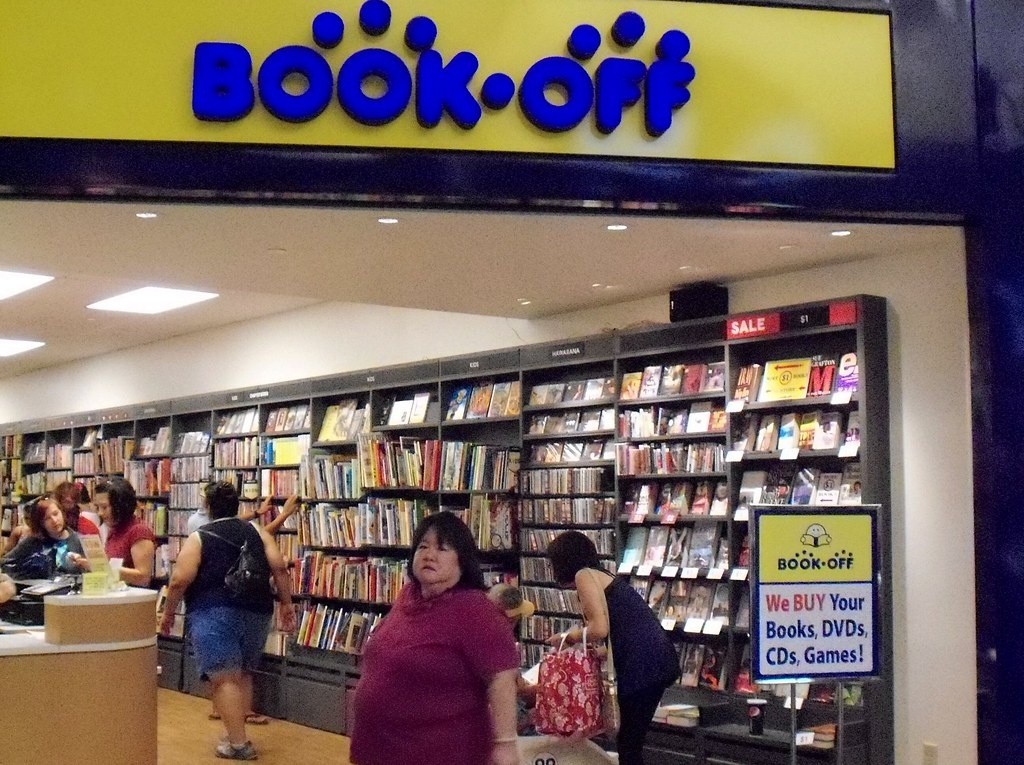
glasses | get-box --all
[35,495,49,505]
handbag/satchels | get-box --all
[535,626,606,739]
[601,678,621,734]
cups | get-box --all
[747,699,767,735]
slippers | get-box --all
[245,714,266,724]
[209,714,221,720]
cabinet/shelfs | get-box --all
[0,293,894,765]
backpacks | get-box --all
[199,522,269,599]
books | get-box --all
[153,352,861,748]
[0,426,152,481]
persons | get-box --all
[484,583,542,736]
[545,531,681,765]
[158,480,298,761]
[185,487,299,725]
[351,513,518,765]
[95,477,154,588]
[1,571,16,605]
[1,482,101,596]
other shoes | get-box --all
[221,735,230,741]
[217,741,256,760]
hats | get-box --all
[486,583,534,617]
